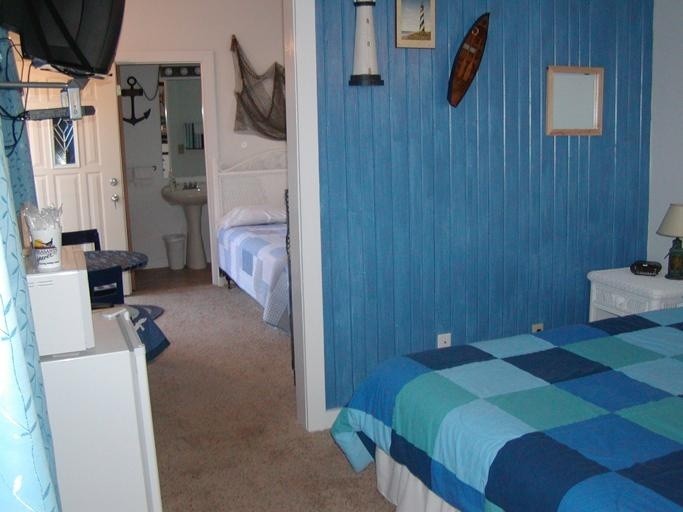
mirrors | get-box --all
[156,64,209,182]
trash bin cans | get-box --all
[162,233,186,270]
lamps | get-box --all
[654,200,683,279]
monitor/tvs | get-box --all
[0,0,126,79]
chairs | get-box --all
[62,229,126,309]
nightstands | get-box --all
[587,265,683,329]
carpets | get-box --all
[124,303,165,322]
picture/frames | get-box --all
[546,66,607,138]
[394,0,437,51]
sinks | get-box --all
[162,186,207,204]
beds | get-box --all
[331,304,683,512]
[211,144,293,338]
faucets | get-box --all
[188,182,194,189]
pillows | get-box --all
[217,205,289,229]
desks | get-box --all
[82,249,149,271]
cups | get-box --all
[30,231,62,271]
